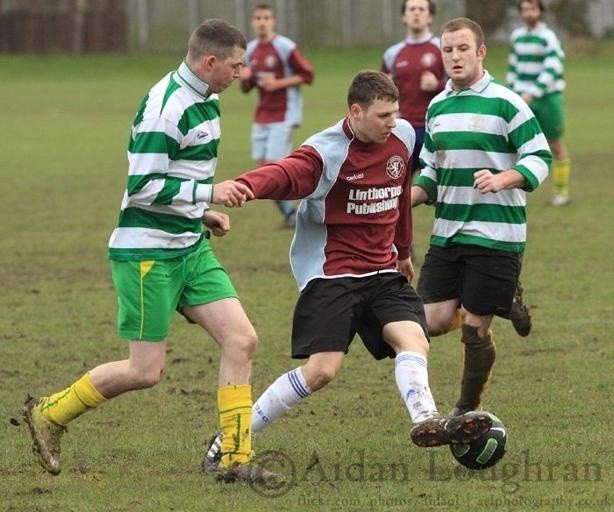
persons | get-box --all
[202,71,493,477]
[380,0,450,176]
[505,1,573,208]
[238,5,315,231]
[411,20,553,418]
[24,18,288,486]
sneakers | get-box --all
[552,194,574,207]
[201,432,286,485]
[21,397,63,476]
[408,412,493,448]
[502,281,533,338]
[280,211,296,228]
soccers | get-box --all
[449,411,507,470]
